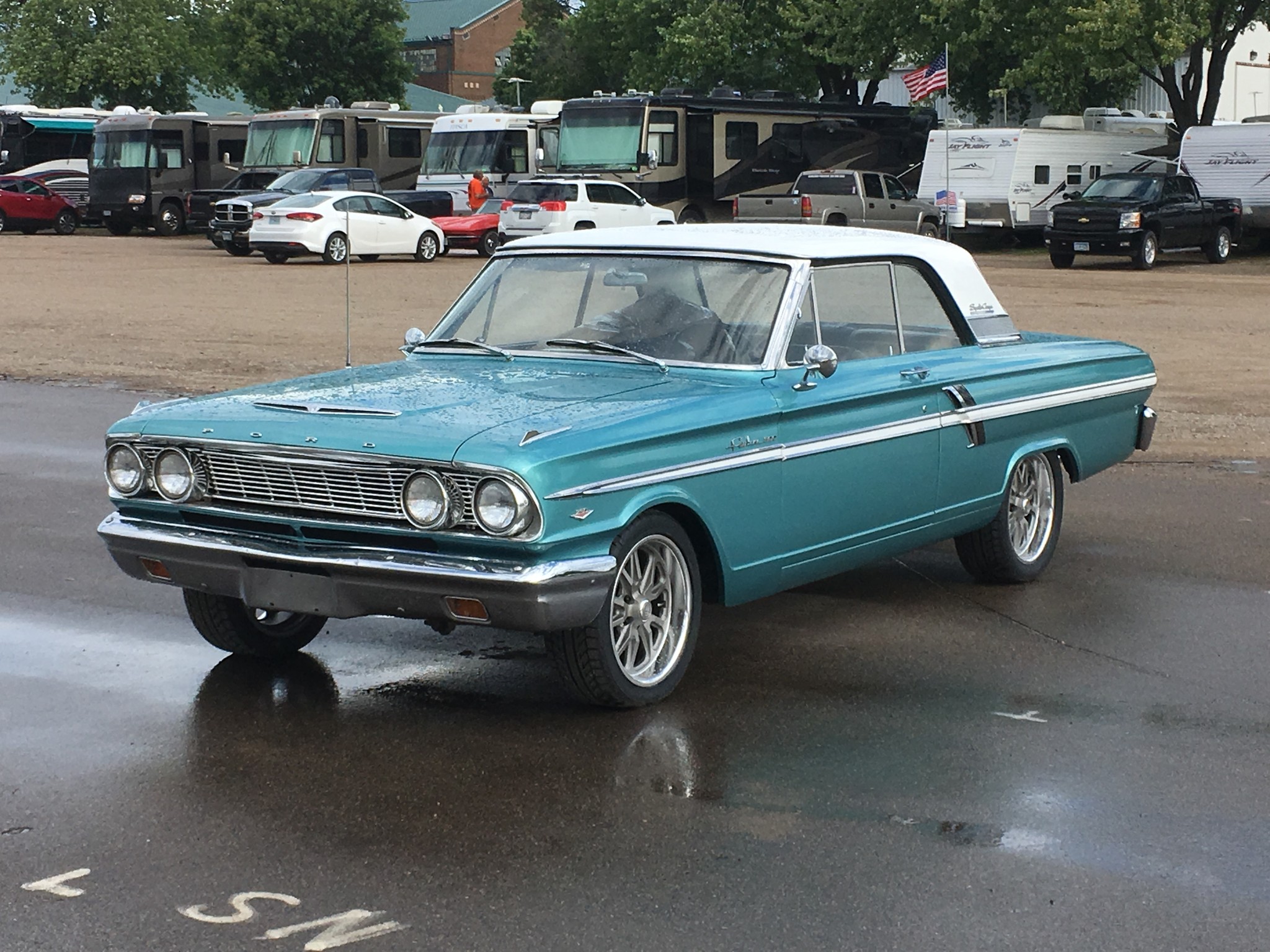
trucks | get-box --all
[537,85,939,226]
[416,101,566,217]
[88,105,252,238]
[0,104,111,225]
[223,95,462,192]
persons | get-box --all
[468,169,491,215]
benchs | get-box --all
[785,322,960,363]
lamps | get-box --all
[1250,51,1257,60]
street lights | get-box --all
[507,77,524,106]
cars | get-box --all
[95,222,1158,711]
[248,190,445,265]
[0,175,82,235]
[430,198,506,259]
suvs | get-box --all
[498,173,676,272]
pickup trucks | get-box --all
[1043,172,1243,270]
[733,169,941,240]
[186,169,289,249]
[206,167,454,256]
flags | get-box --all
[901,51,947,102]
